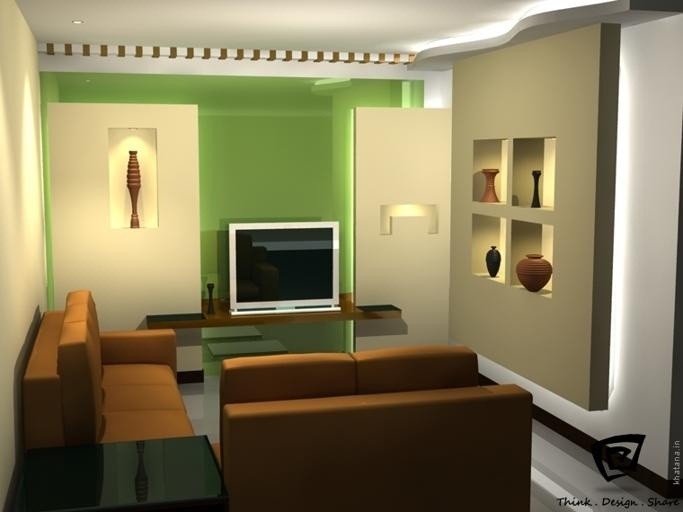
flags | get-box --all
[531,170,541,208]
[127,150,141,229]
[486,246,501,277]
[516,254,553,293]
[480,169,500,202]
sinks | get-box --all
[22,289,195,451]
[210,344,533,512]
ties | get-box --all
[226,221,343,316]
